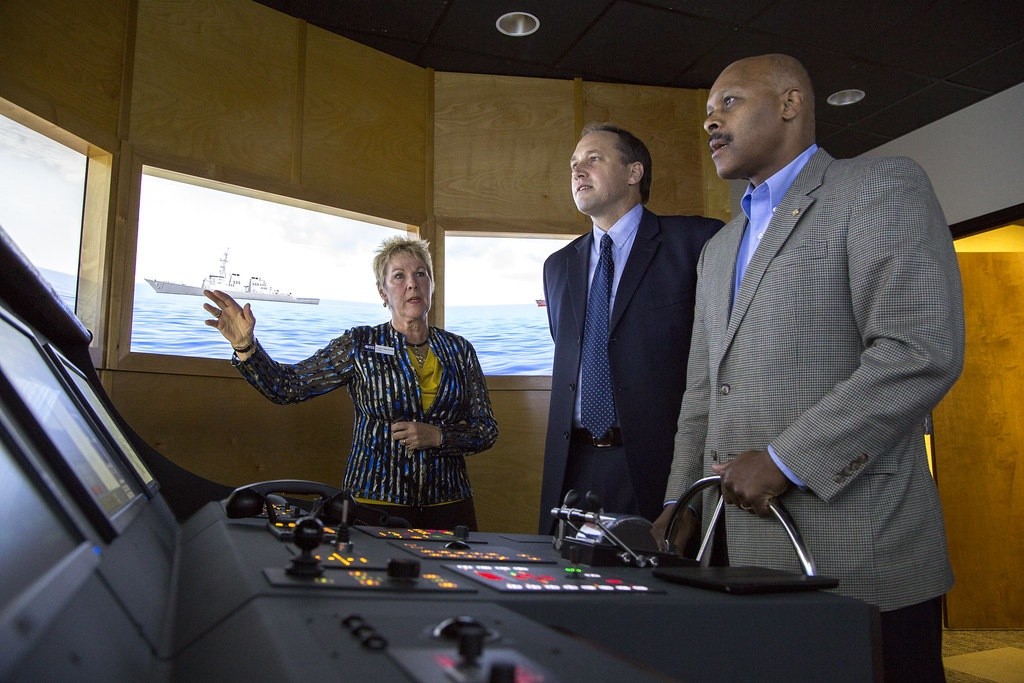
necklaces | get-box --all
[404,333,430,366]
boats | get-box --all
[144,250,321,305]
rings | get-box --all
[403,438,407,445]
[216,310,223,318]
[740,502,753,510]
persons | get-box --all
[201,236,501,533]
[645,49,968,681]
[537,118,733,540]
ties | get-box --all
[579,233,618,443]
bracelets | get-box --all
[230,335,256,354]
